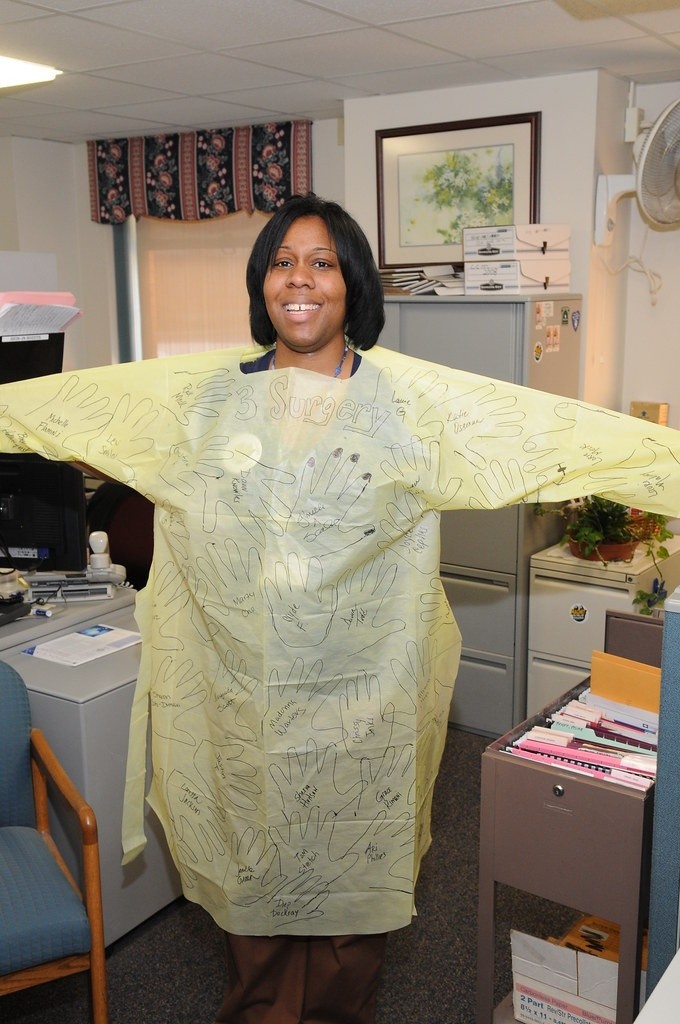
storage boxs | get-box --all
[509,915,646,1024]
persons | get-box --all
[0,196,680,1024]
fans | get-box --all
[594,98,680,247]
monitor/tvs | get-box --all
[1,453,86,571]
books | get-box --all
[499,649,661,792]
[378,265,464,296]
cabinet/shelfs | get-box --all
[378,292,580,738]
[475,610,665,1024]
[525,534,679,720]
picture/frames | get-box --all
[375,111,543,270]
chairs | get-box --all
[0,663,107,1024]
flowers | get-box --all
[534,496,666,615]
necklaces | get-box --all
[272,343,349,378]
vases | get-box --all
[568,539,642,561]
